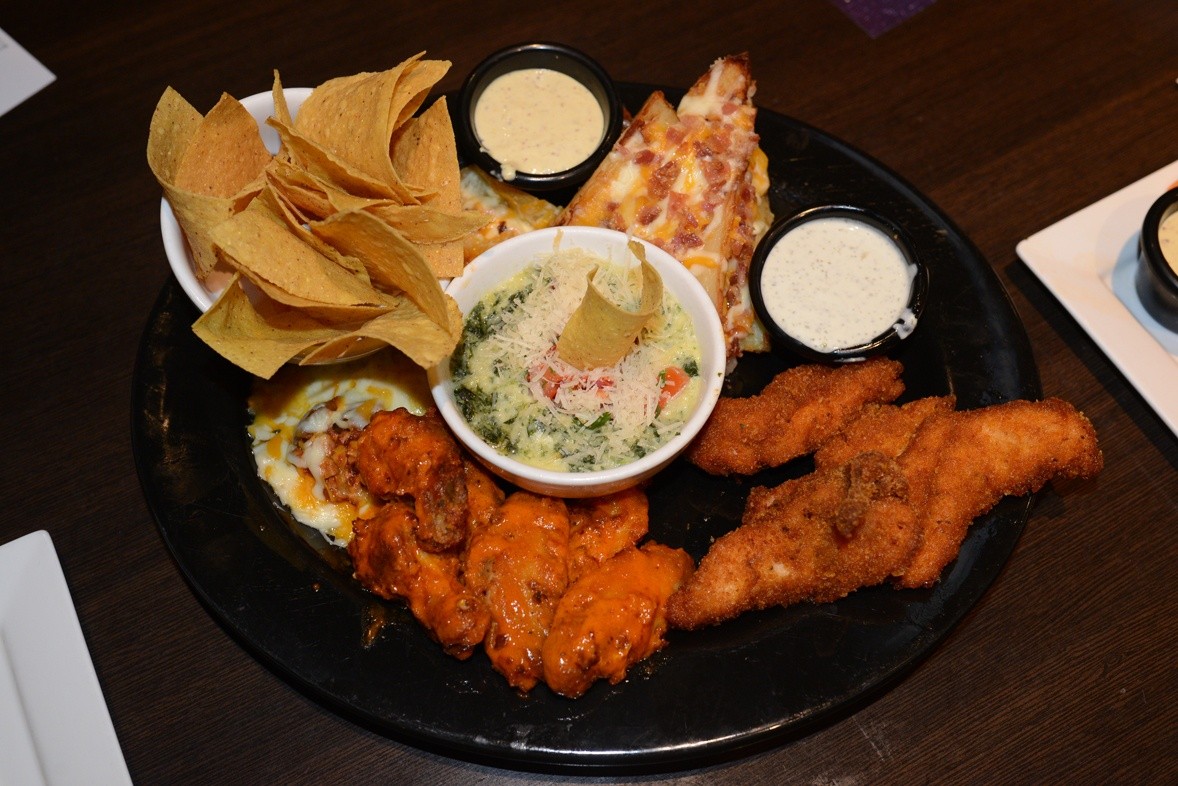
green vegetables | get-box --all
[449,285,700,475]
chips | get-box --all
[145,51,488,378]
[558,239,664,370]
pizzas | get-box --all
[547,48,779,365]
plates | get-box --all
[125,83,1046,779]
[1014,159,1178,440]
[0,528,134,786]
[1,29,58,118]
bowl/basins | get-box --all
[746,204,932,363]
[159,85,454,365]
[461,43,624,203]
[429,224,727,499]
[1140,186,1178,314]
